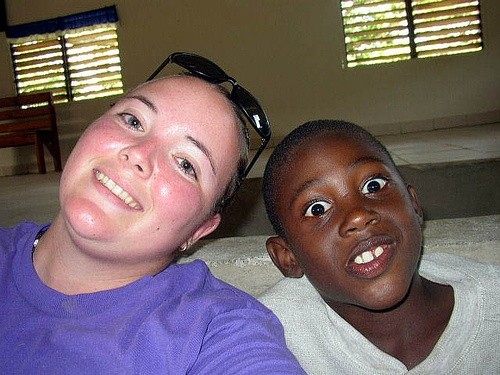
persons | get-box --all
[0,51,306,375]
[256,119,500,375]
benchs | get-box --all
[0,91,62,174]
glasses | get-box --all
[145,52,272,206]
